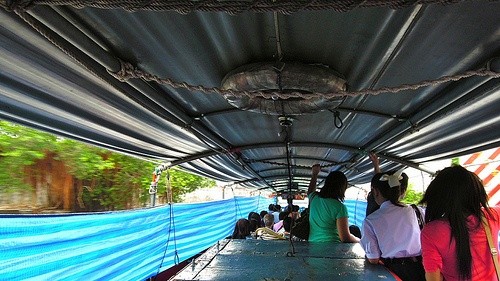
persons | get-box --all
[232,205,362,243]
[360,151,500,281]
[307,163,361,243]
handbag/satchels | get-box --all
[291,191,318,240]
